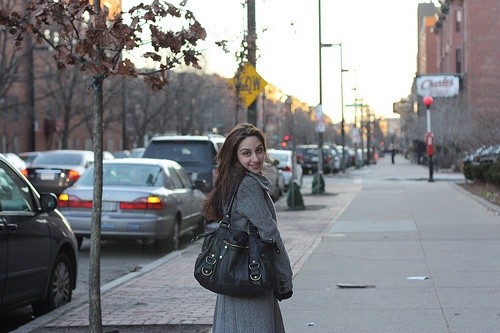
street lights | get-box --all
[423,94,436,183]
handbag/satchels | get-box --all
[192,174,280,298]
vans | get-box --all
[0,154,78,317]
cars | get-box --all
[23,150,114,197]
[263,157,284,202]
[295,144,355,175]
[57,158,208,256]
[266,149,302,189]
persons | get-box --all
[388,145,397,164]
[200,123,295,333]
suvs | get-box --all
[141,135,228,194]
